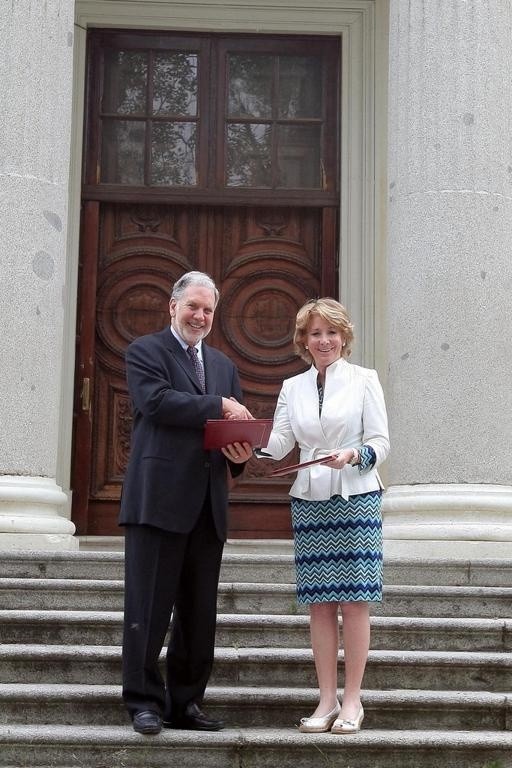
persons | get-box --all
[116,270,262,735]
[219,295,393,736]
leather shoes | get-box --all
[133,710,162,734]
[300,697,341,732]
[330,702,364,734]
[163,703,224,731]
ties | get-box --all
[187,347,206,392]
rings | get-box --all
[234,455,240,459]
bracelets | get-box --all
[349,448,359,466]
